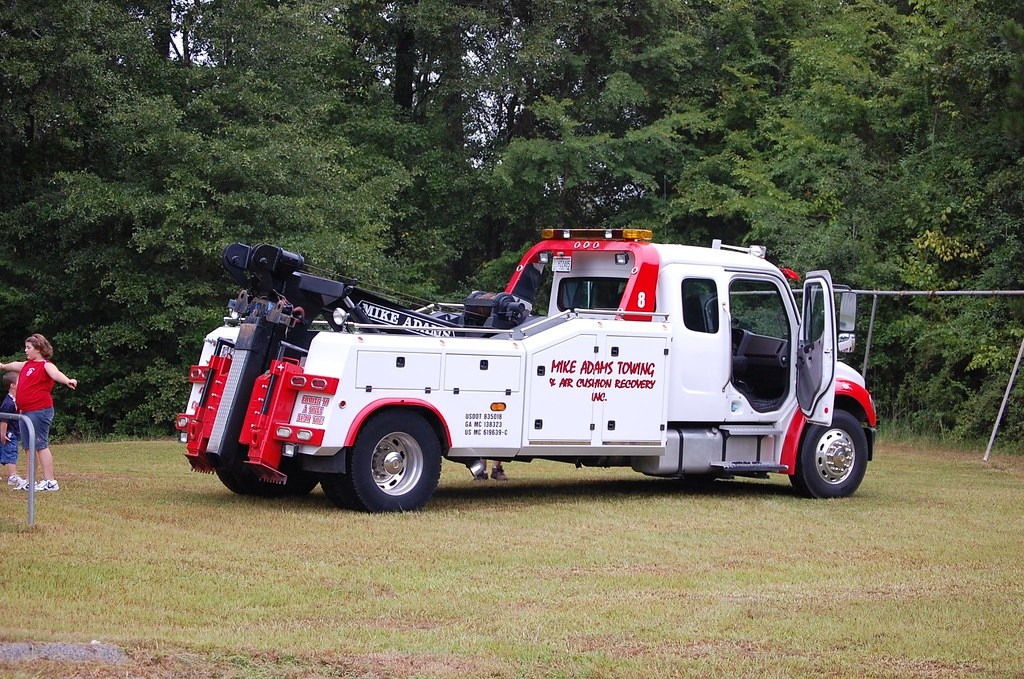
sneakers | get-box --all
[14,480,36,491]
[8,473,25,485]
[32,480,60,492]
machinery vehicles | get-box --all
[173,228,878,514]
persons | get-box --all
[474,458,509,480]
[0,334,77,492]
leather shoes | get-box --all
[473,473,488,480]
[491,466,507,480]
